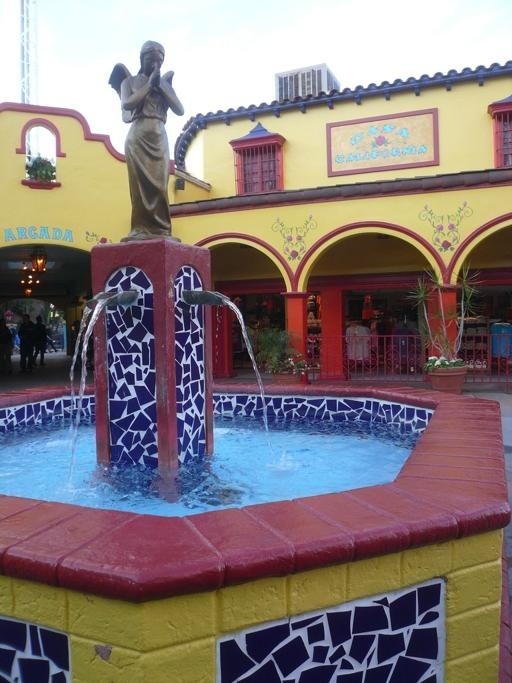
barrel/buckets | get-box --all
[490,325,512,357]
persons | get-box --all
[119,39,186,240]
[1,311,59,378]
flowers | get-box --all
[404,265,486,371]
[254,328,312,376]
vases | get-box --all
[271,366,303,384]
[427,364,467,393]
